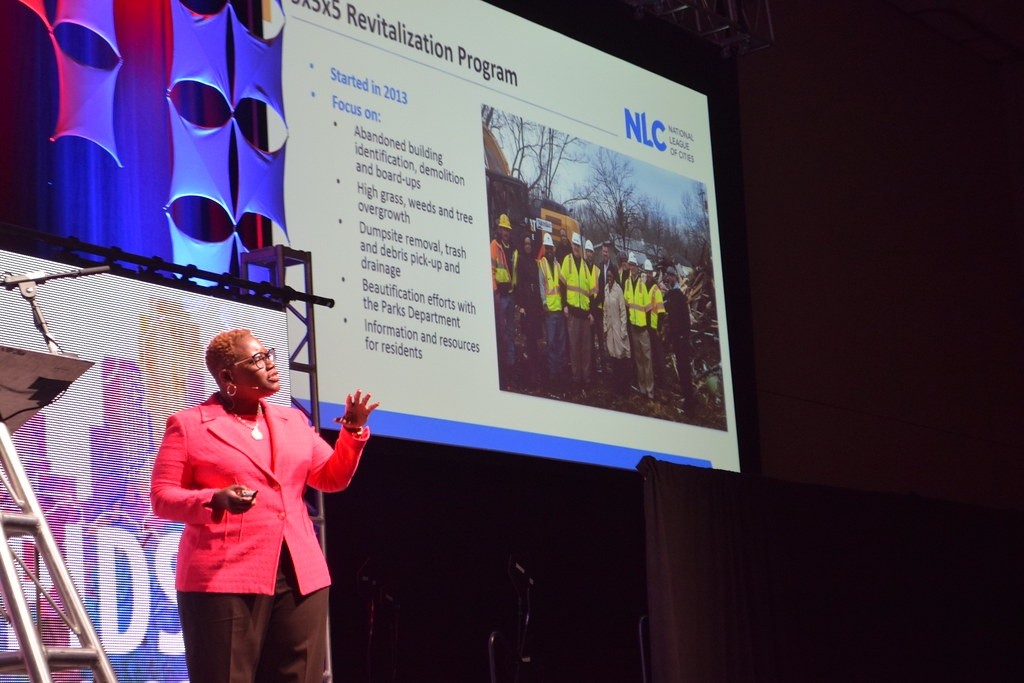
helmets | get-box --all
[498,214,512,230]
[644,260,653,270]
[543,232,554,246]
[585,240,593,252]
[628,252,638,266]
[572,233,581,246]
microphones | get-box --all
[251,386,258,389]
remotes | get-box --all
[240,489,259,498]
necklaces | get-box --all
[224,401,263,441]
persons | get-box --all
[490,213,701,418]
[147,326,381,683]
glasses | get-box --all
[230,348,276,369]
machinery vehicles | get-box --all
[482,103,583,261]
